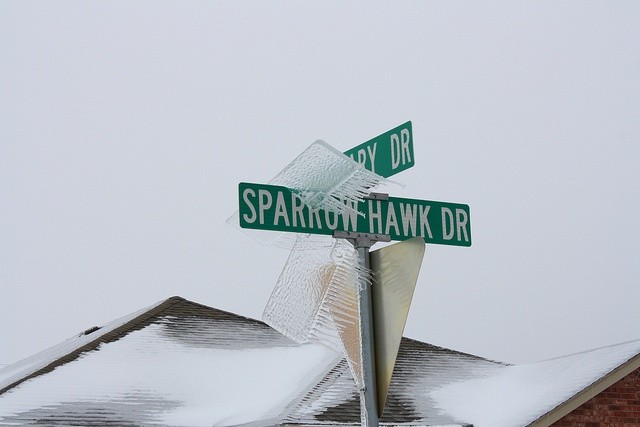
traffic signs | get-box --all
[236,181,473,248]
[342,121,415,177]
